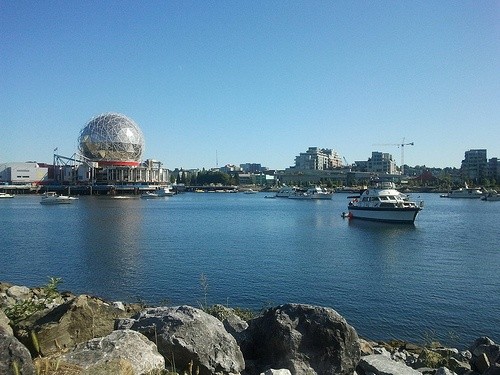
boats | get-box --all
[39,194,80,205]
[244,189,259,194]
[440,181,500,201]
[141,191,158,199]
[276,184,334,198]
[152,189,176,196]
[111,195,137,199]
[194,188,239,193]
[41,192,58,199]
[347,185,424,224]
[0,193,16,198]
[264,195,276,198]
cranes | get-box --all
[373,136,414,175]
